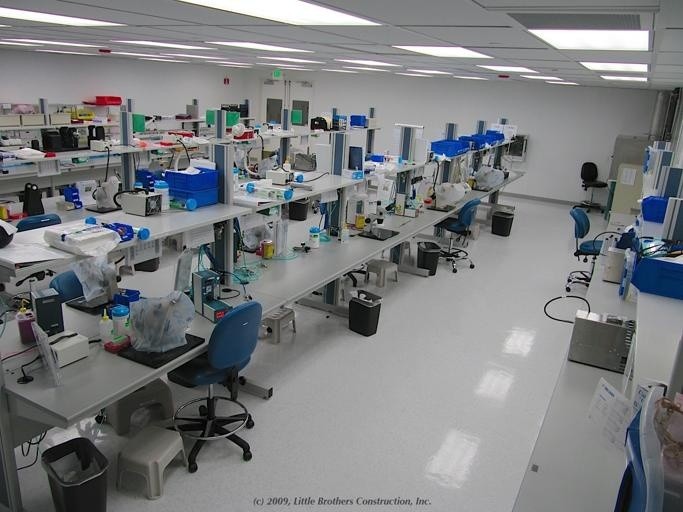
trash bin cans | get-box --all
[289,199,310,221]
[417,241,441,276]
[41,438,110,512]
[492,211,514,237]
[349,290,383,337]
[134,257,160,272]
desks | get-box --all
[512,210,681,512]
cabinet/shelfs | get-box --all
[0,96,526,512]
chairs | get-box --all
[565,208,603,293]
[572,162,608,214]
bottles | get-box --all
[170,196,197,210]
[155,181,169,211]
[15,307,40,343]
[341,225,350,244]
[245,182,291,199]
[101,302,132,352]
[309,226,320,248]
